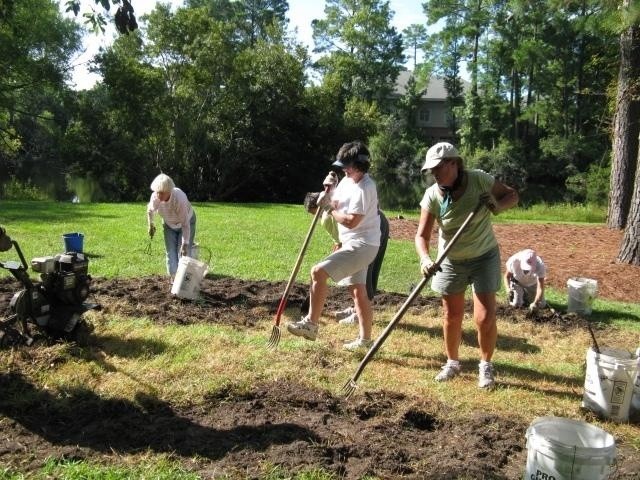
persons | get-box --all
[413,141,519,390]
[145,173,197,290]
[285,142,382,352]
[303,189,390,326]
[504,249,546,313]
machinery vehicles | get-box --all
[0,232,103,350]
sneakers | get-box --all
[477,361,496,390]
[333,305,357,321]
[285,316,321,341]
[343,336,375,351]
[435,363,461,382]
[338,313,359,325]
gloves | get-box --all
[321,171,339,189]
[316,191,334,213]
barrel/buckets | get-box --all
[61,232,84,253]
[522,418,616,480]
[170,245,211,300]
[580,346,639,424]
[566,278,597,314]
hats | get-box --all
[331,153,369,169]
[520,249,539,270]
[420,141,459,176]
